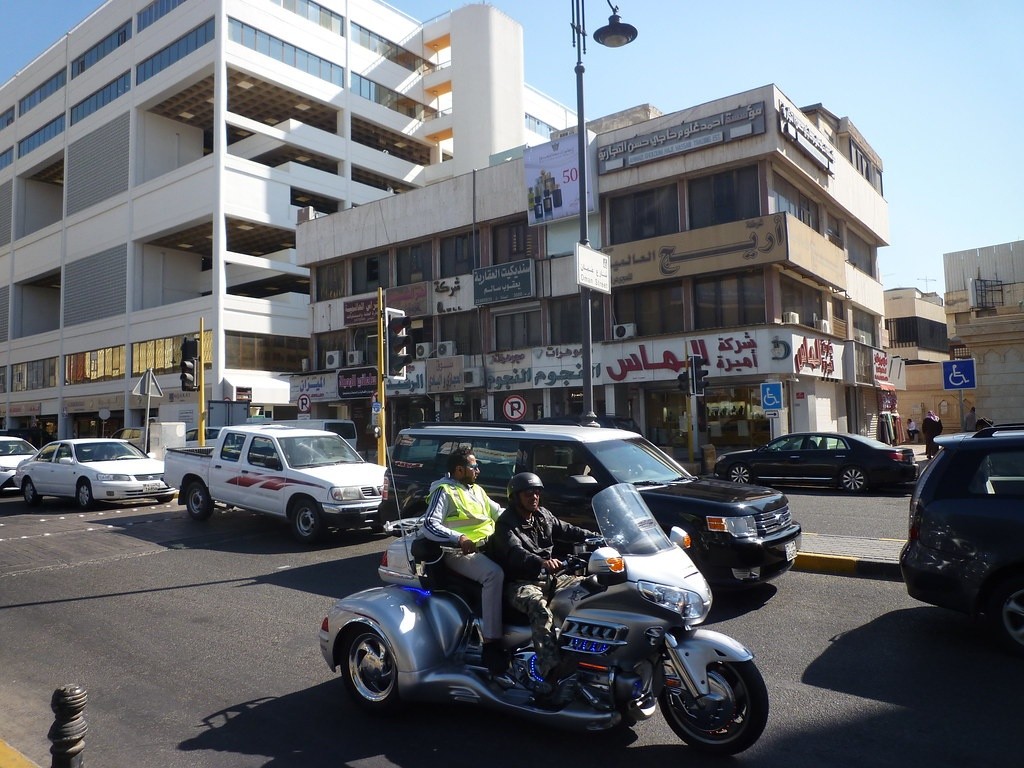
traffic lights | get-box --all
[385,306,413,382]
[179,359,197,392]
[677,373,688,390]
[182,341,200,359]
[692,354,710,396]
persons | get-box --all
[906,419,919,441]
[964,407,978,431]
[922,410,943,459]
[490,472,602,700]
[975,416,994,432]
[426,449,507,673]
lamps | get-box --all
[785,374,800,382]
[821,377,839,384]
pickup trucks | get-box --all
[162,424,389,543]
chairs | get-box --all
[572,448,591,475]
[254,446,274,464]
[808,440,817,449]
[535,447,562,484]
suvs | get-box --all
[898,421,1024,654]
[375,417,803,601]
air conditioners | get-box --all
[783,312,800,324]
[464,367,485,387]
[855,335,866,344]
[613,323,637,340]
[416,342,433,359]
[437,341,456,356]
[816,320,830,333]
[348,350,363,366]
[326,351,343,369]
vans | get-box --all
[264,417,359,460]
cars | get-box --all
[0,435,40,493]
[7,427,55,447]
[713,432,921,494]
[12,438,178,509]
[182,427,225,448]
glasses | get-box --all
[466,464,479,470]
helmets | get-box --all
[507,472,544,499]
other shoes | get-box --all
[482,642,511,662]
[544,669,571,702]
[927,456,931,460]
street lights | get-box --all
[570,0,641,429]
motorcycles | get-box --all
[318,484,771,755]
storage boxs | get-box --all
[386,529,424,575]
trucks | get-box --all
[108,421,186,457]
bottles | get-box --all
[544,190,552,213]
[535,171,555,196]
[528,188,535,210]
[535,197,543,218]
[553,185,562,208]
